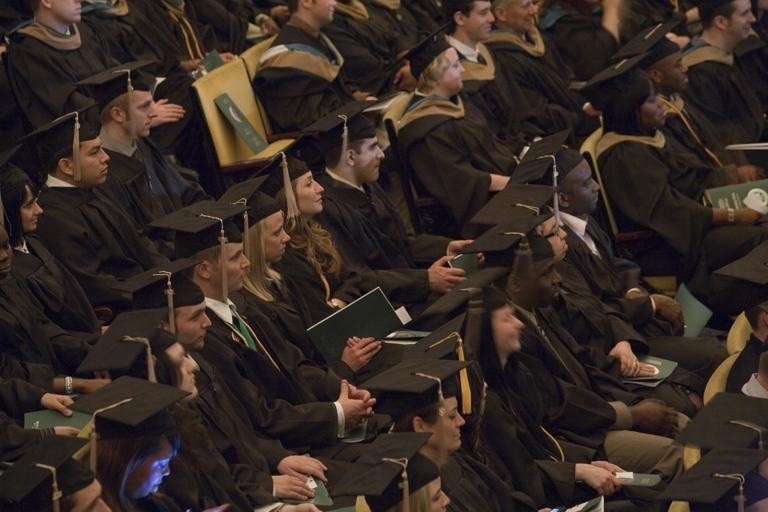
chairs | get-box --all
[192,36,445,237]
[452,342,473,416]
[666,311,750,511]
[579,126,646,242]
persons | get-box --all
[1,1,768,512]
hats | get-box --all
[0,21,768,512]
